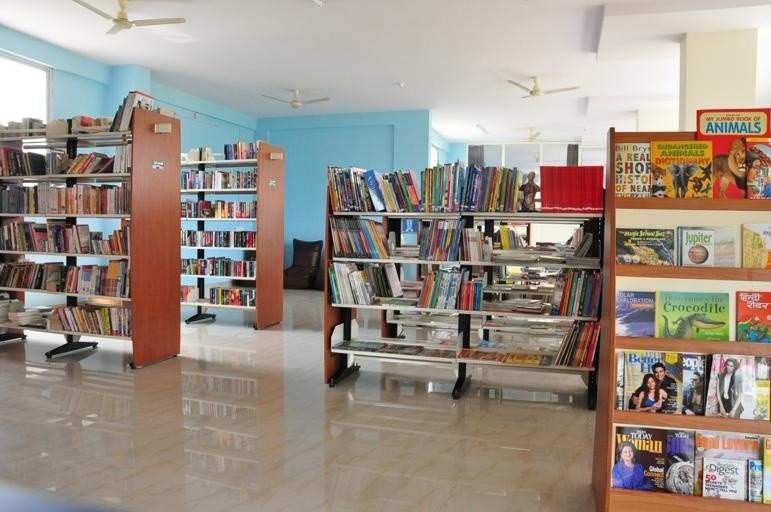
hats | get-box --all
[728,358,739,369]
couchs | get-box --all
[284,238,323,290]
[314,268,324,290]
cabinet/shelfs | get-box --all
[321,160,602,410]
[0,107,182,369]
[122,139,284,331]
[591,126,771,511]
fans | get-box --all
[507,75,581,100]
[73,0,186,35]
[518,127,542,143]
[260,88,332,109]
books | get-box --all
[735,291,771,344]
[328,216,603,266]
[553,320,601,369]
[327,262,603,319]
[697,107,771,200]
[676,225,736,267]
[746,137,771,200]
[704,353,771,421]
[223,140,259,159]
[0,90,153,138]
[741,223,771,270]
[456,348,550,368]
[210,287,256,307]
[0,260,131,306]
[702,457,748,502]
[181,197,256,219]
[747,460,763,504]
[615,290,656,338]
[180,166,257,189]
[180,229,256,248]
[695,430,771,497]
[327,163,418,213]
[0,182,130,214]
[762,438,771,505]
[1,298,133,338]
[180,285,199,302]
[615,143,651,199]
[0,146,133,174]
[419,158,519,213]
[656,292,730,342]
[623,351,683,414]
[650,141,713,200]
[611,423,695,497]
[615,228,675,267]
[614,348,706,420]
[681,229,715,267]
[331,340,456,359]
[540,165,604,214]
[181,256,254,278]
[0,217,130,255]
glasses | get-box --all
[750,166,759,171]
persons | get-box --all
[631,363,677,413]
[716,358,744,419]
[516,172,538,212]
[612,441,657,490]
[636,374,663,413]
[757,357,770,381]
